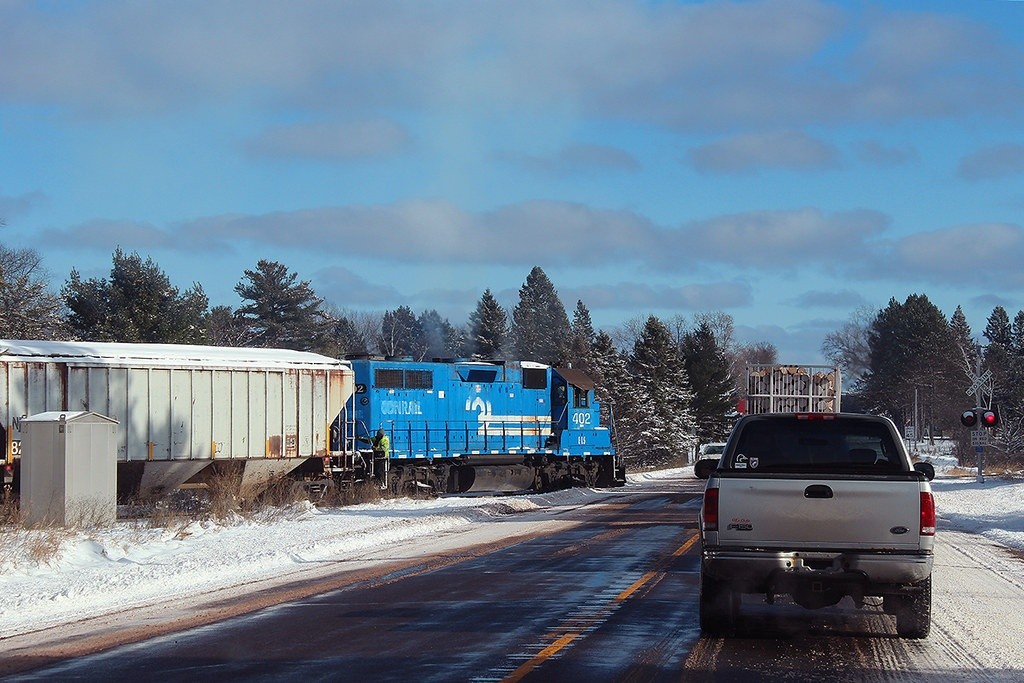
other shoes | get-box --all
[380,484,388,490]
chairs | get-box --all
[847,446,878,464]
[751,444,782,463]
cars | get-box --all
[696,441,727,461]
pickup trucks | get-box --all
[693,410,939,640]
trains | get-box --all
[0,332,628,514]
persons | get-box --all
[357,427,390,491]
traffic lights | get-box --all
[960,410,977,427]
[981,410,999,427]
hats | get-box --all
[378,429,384,435]
